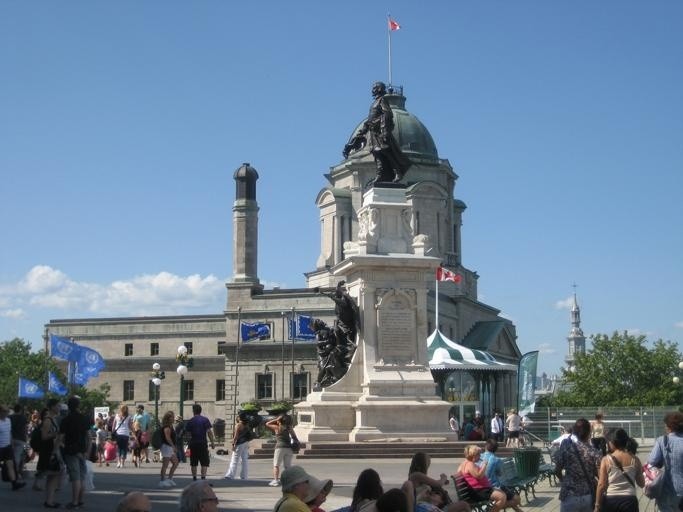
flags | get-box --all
[388,16,401,32]
[288,320,313,342]
[241,321,269,343]
[435,266,460,283]
[295,314,315,337]
[15,334,105,398]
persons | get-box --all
[359,82,403,182]
[313,281,359,392]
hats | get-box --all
[304,474,335,507]
[174,414,183,423]
[280,465,314,491]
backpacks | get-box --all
[151,428,163,452]
[29,427,44,454]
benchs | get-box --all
[333,443,561,512]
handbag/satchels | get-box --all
[110,432,117,441]
[639,461,668,503]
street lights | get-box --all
[635,406,649,444]
[151,361,166,432]
[551,407,564,438]
[673,361,683,390]
[176,345,194,419]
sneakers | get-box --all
[268,479,281,487]
[96,457,151,470]
[9,479,89,510]
[166,478,177,486]
[158,480,172,488]
